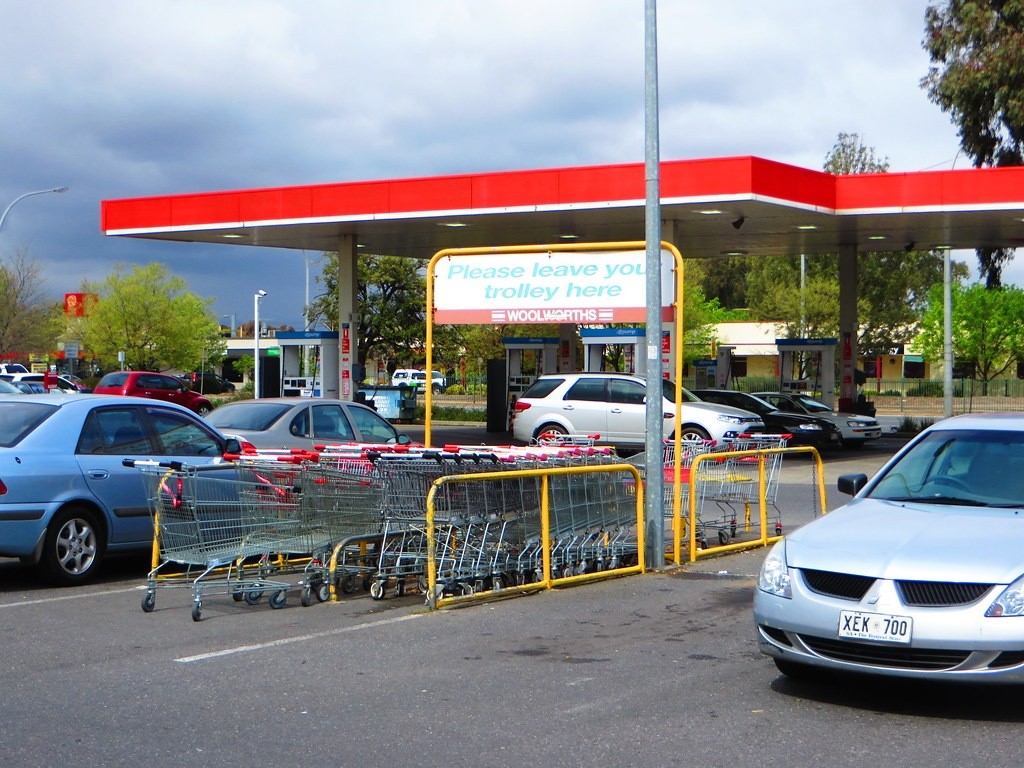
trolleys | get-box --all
[142,434,792,623]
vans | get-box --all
[392,369,447,396]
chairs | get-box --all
[940,454,1003,498]
[312,416,345,440]
[109,426,148,453]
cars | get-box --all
[691,388,842,460]
[750,410,1024,716]
[95,371,214,418]
[201,397,427,455]
[752,391,881,451]
[0,363,90,396]
[1,392,274,585]
[514,373,765,469]
